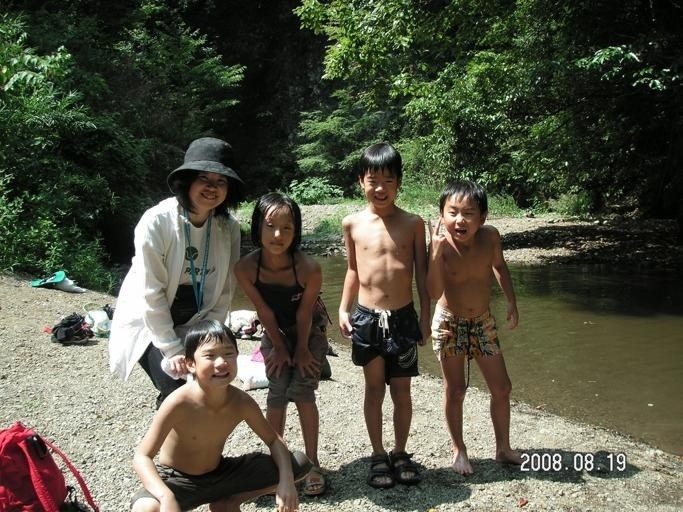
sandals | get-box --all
[301,454,326,495]
[367,449,395,488]
[389,451,422,486]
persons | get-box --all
[108,136,248,411]
[426,179,534,476]
[339,140,432,485]
[233,192,339,499]
[132,318,316,511]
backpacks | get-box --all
[0,420,99,512]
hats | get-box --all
[168,137,249,196]
[31,270,65,287]
